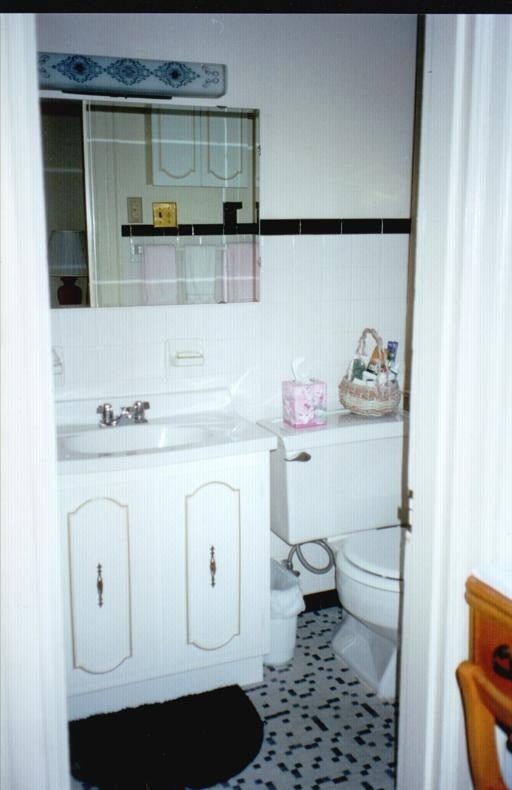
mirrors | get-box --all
[39,99,260,306]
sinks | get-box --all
[66,425,209,453]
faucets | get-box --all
[98,403,134,428]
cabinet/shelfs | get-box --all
[59,450,269,722]
[150,104,252,189]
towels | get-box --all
[138,242,256,303]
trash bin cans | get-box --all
[264,561,301,668]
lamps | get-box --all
[48,230,88,304]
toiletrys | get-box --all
[350,337,401,387]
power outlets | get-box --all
[152,202,177,228]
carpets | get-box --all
[69,684,264,789]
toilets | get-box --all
[256,407,400,704]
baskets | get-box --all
[339,328,401,418]
